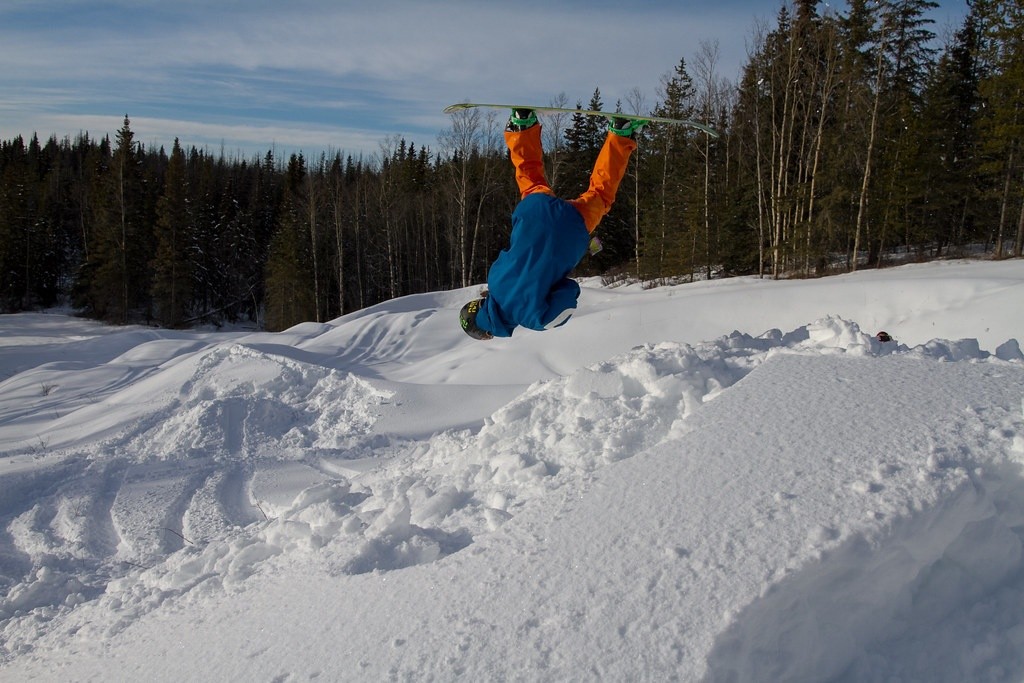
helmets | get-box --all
[459,298,495,341]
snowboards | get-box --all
[443,104,720,138]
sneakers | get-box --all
[512,109,536,131]
[611,118,632,138]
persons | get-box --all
[459,107,650,340]
[876,331,889,342]
